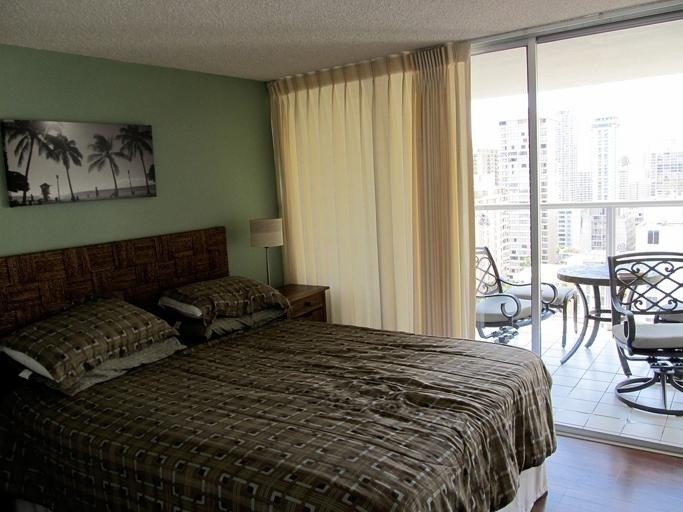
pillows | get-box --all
[174,307,284,343]
[157,275,291,323]
[1,292,181,382]
[46,336,187,398]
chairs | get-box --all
[469,245,559,346]
[605,249,683,417]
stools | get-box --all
[504,285,579,348]
[652,300,683,326]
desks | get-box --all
[556,265,664,379]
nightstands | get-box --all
[268,284,330,324]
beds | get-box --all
[1,226,558,512]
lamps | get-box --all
[248,217,285,284]
